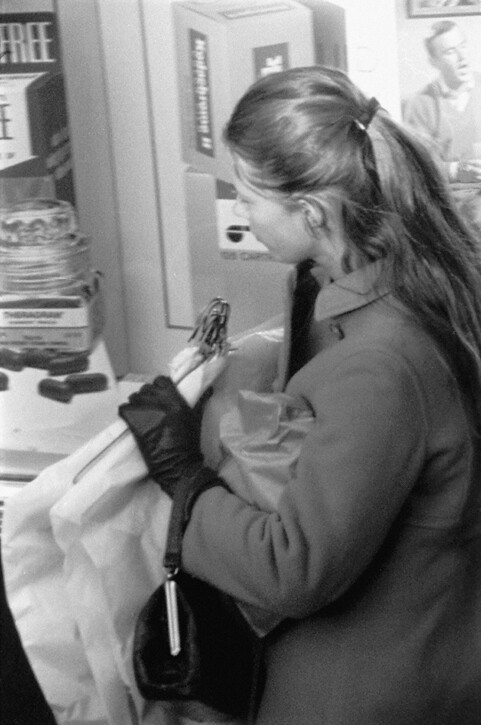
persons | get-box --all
[401,20,481,204]
[118,66,481,725]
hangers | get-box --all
[74,296,237,481]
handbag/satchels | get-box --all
[133,466,269,722]
[214,390,315,517]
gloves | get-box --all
[118,376,215,499]
